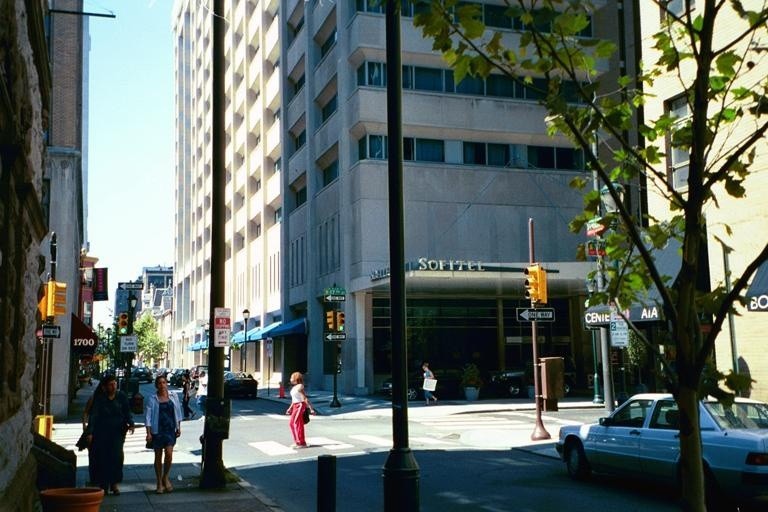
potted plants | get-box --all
[461,362,483,402]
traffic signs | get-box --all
[117,282,144,291]
[586,216,608,256]
[516,307,556,323]
[43,325,60,338]
[324,286,346,303]
[324,333,347,341]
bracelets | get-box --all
[289,404,293,409]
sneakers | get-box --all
[111,484,121,495]
[290,442,308,449]
[157,479,173,494]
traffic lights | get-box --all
[37,280,67,321]
[118,311,131,334]
[326,310,345,333]
[523,264,549,305]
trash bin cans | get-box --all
[120,378,139,409]
[31,430,76,492]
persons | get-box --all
[80,381,105,434]
[181,368,208,421]
[421,362,438,405]
[285,371,316,448]
[82,374,134,496]
[144,375,182,494]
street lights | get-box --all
[602,178,629,406]
[127,294,137,394]
[242,308,250,372]
[584,271,603,404]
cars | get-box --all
[487,354,578,396]
[555,393,768,502]
[153,367,258,399]
[381,366,487,401]
[133,366,153,383]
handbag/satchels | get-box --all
[422,378,437,392]
